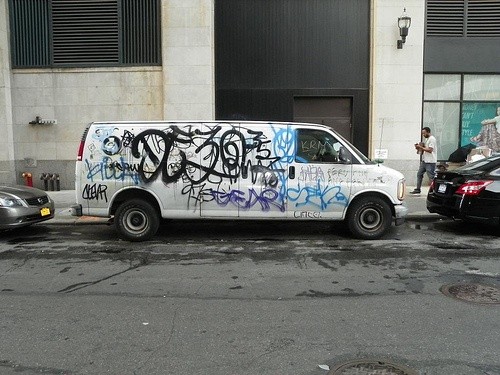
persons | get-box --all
[410,127,437,194]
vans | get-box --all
[68,118,407,240]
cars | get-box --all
[426,153,500,229]
[0,182,55,234]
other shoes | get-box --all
[409,189,420,193]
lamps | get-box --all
[397,7,411,49]
